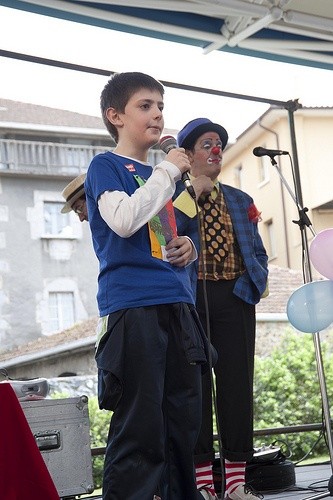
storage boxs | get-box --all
[18,395,94,496]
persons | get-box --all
[84,71,208,500]
[61,173,88,222]
[177,118,268,500]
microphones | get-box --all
[160,136,196,197]
[252,147,289,156]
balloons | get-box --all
[287,279,333,333]
[310,228,333,281]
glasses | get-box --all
[75,199,86,217]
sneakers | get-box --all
[199,484,217,500]
[225,479,265,500]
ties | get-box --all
[203,192,229,263]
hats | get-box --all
[61,173,86,214]
[176,118,228,151]
